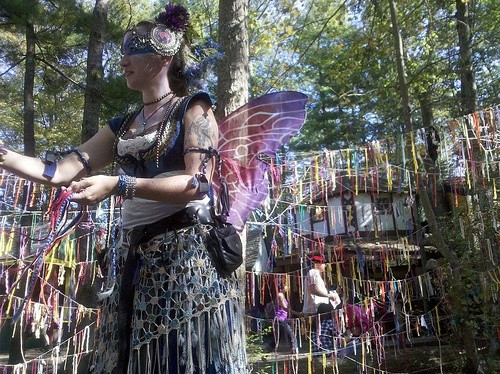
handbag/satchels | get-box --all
[209,224,243,275]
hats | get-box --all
[307,256,327,266]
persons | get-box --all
[0,3,249,374]
[272,278,297,354]
[302,252,343,359]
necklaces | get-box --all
[142,91,176,127]
[109,93,178,175]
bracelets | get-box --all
[1,142,6,165]
[183,146,230,216]
[43,149,92,177]
[118,175,137,199]
